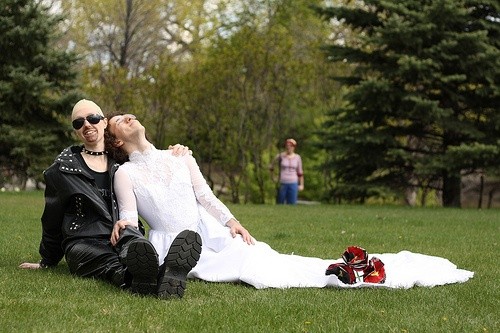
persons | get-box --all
[103,112,475,290]
[271,138,304,205]
[15,98,202,302]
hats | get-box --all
[285,138,297,147]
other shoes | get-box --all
[127,229,202,299]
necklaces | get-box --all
[81,147,110,158]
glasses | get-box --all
[72,114,105,129]
[113,114,137,133]
